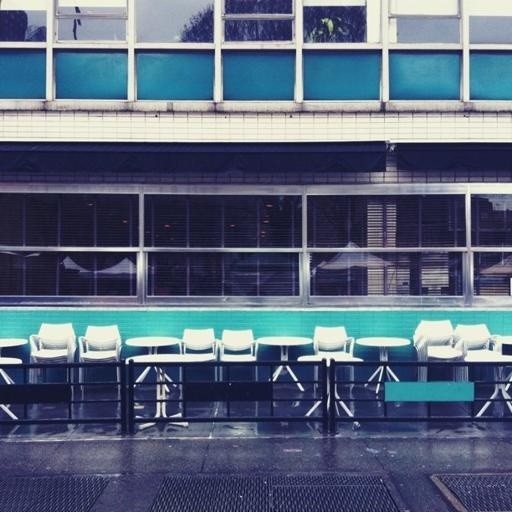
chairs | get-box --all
[313,326,355,388]
[415,320,494,388]
[181,328,256,383]
[28,323,122,389]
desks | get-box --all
[0,338,29,385]
[125,336,179,388]
[357,337,411,394]
[258,336,314,391]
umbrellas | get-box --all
[59,254,90,274]
[479,253,511,276]
[315,241,390,270]
[93,259,136,279]
